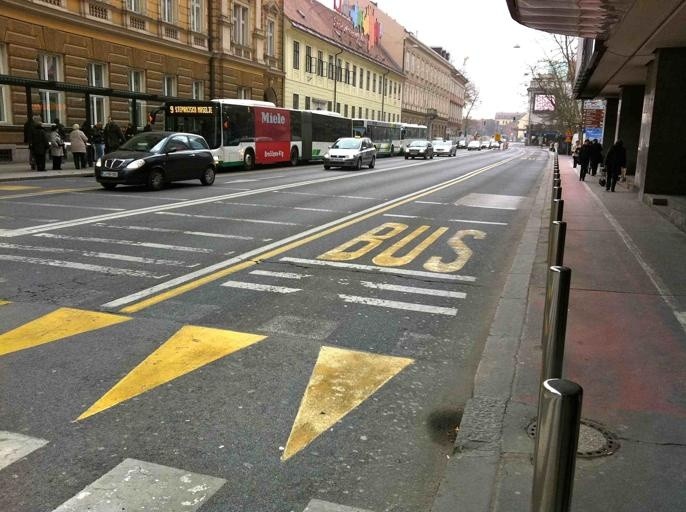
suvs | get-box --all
[324,136,377,172]
[94,130,216,191]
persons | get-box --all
[604,140,627,192]
[20,114,151,172]
[571,137,603,182]
[548,140,560,155]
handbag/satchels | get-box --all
[599,178,605,185]
[56,140,64,145]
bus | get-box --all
[352,118,403,157]
[150,96,354,171]
[401,122,428,152]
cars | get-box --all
[491,140,499,148]
[430,137,445,145]
[405,141,434,160]
[468,141,481,151]
[480,141,491,149]
[433,140,457,157]
[456,140,468,148]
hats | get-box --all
[72,123,79,129]
